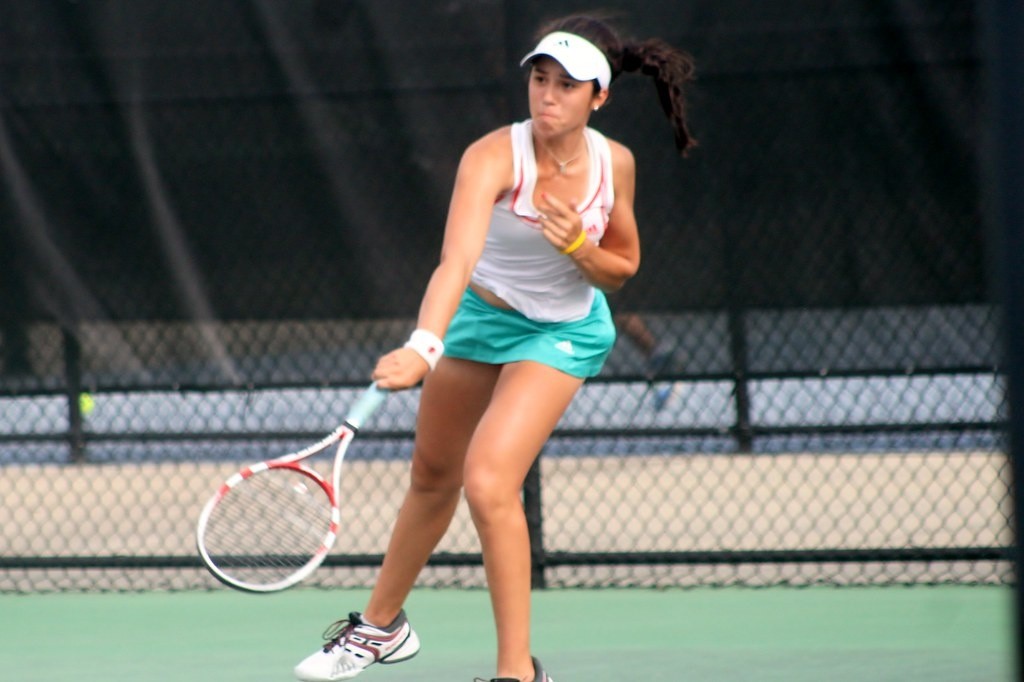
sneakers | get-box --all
[473,656,554,682]
[294,611,420,682]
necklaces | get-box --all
[531,123,587,178]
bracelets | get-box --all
[403,329,445,372]
[558,231,587,255]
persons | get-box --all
[603,292,677,411]
[294,14,698,682]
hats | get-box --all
[519,29,612,89]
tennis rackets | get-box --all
[194,380,391,595]
